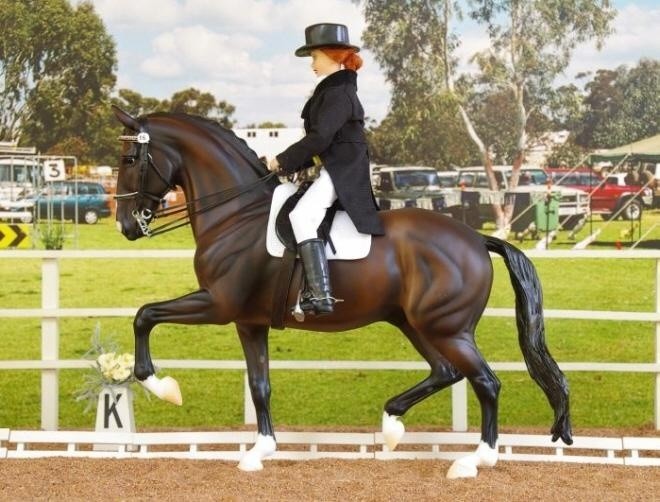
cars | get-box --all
[9,180,112,224]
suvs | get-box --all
[372,163,654,230]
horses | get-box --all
[106,100,575,481]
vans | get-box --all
[0,159,46,211]
